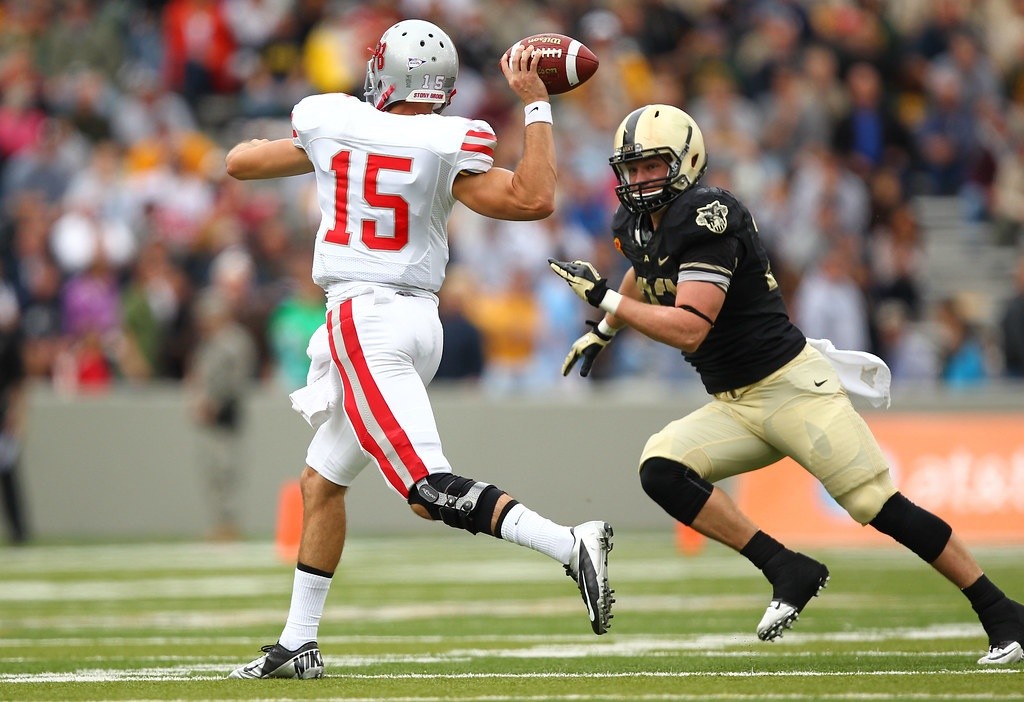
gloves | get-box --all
[561,320,613,377]
[547,257,610,309]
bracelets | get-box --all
[524,101,553,126]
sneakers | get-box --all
[756,552,830,642]
[227,640,325,680]
[563,521,614,635]
[977,600,1024,665]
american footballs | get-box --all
[498,32,601,96]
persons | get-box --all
[547,103,1024,665]
[0,333,25,543]
[185,286,254,540]
[224,18,616,675]
[0,0,1024,386]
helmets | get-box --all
[609,104,708,216]
[363,19,459,114]
